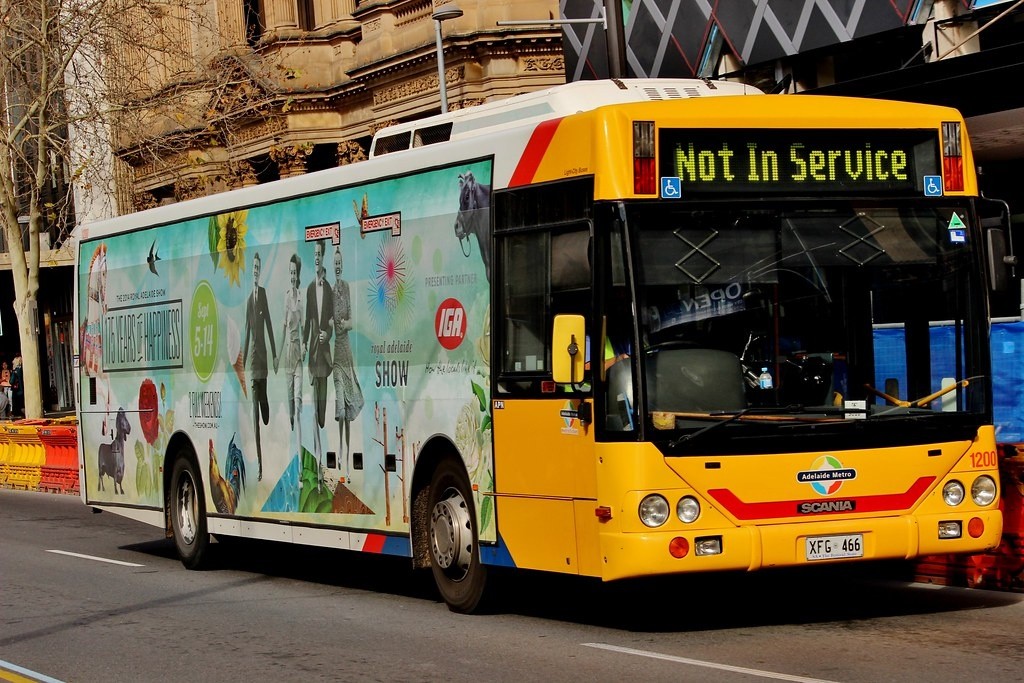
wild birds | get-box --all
[146,238,162,277]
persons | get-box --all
[0,356,24,418]
[276,254,306,489]
[301,241,334,494]
[243,251,276,482]
[327,245,365,483]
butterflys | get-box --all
[351,192,369,240]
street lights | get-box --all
[432,8,464,114]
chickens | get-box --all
[208,432,247,515]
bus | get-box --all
[73,79,1002,613]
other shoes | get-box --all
[298,473,303,487]
[257,471,262,481]
[9,411,12,418]
[347,473,350,484]
[338,456,341,469]
[318,482,324,494]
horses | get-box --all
[454,170,490,282]
[79,239,110,436]
